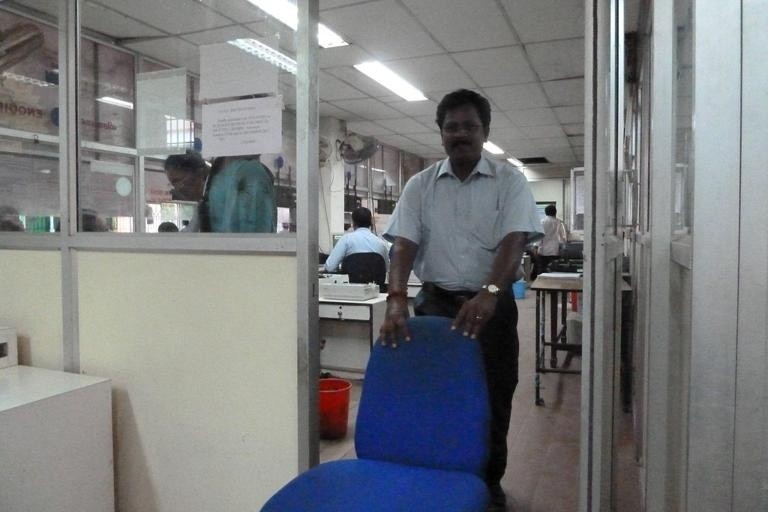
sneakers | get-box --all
[490,485,506,508]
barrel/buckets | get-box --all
[513,280,527,299]
[317,376,353,442]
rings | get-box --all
[476,316,484,320]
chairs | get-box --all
[260,315,491,511]
[342,252,386,293]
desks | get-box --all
[530,273,633,414]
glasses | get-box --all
[439,122,483,136]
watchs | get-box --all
[480,283,505,299]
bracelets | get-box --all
[385,291,408,303]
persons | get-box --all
[199,93,278,234]
[529,204,567,280]
[384,86,546,512]
[324,205,390,274]
[162,150,209,201]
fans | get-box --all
[319,132,379,164]
